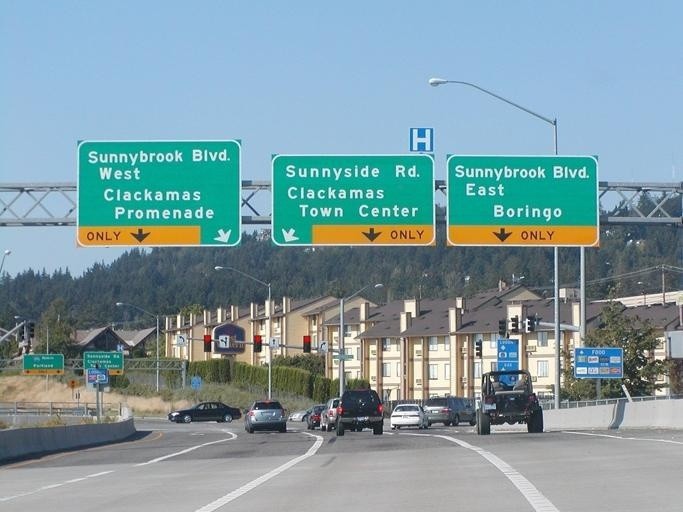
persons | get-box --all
[491,376,500,382]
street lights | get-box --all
[12,315,49,389]
[427,76,562,410]
[214,266,272,398]
[115,302,159,391]
[338,282,384,397]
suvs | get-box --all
[423,396,476,427]
[475,369,542,434]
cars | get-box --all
[70,406,97,417]
[166,401,241,423]
[289,389,384,436]
[242,400,287,433]
[389,403,429,430]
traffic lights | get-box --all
[253,334,262,353]
[302,336,311,353]
[202,334,211,352]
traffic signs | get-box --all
[268,154,437,249]
[444,153,601,247]
[20,354,64,376]
[82,351,124,385]
[573,347,624,379]
[75,138,243,249]
[495,338,520,388]
